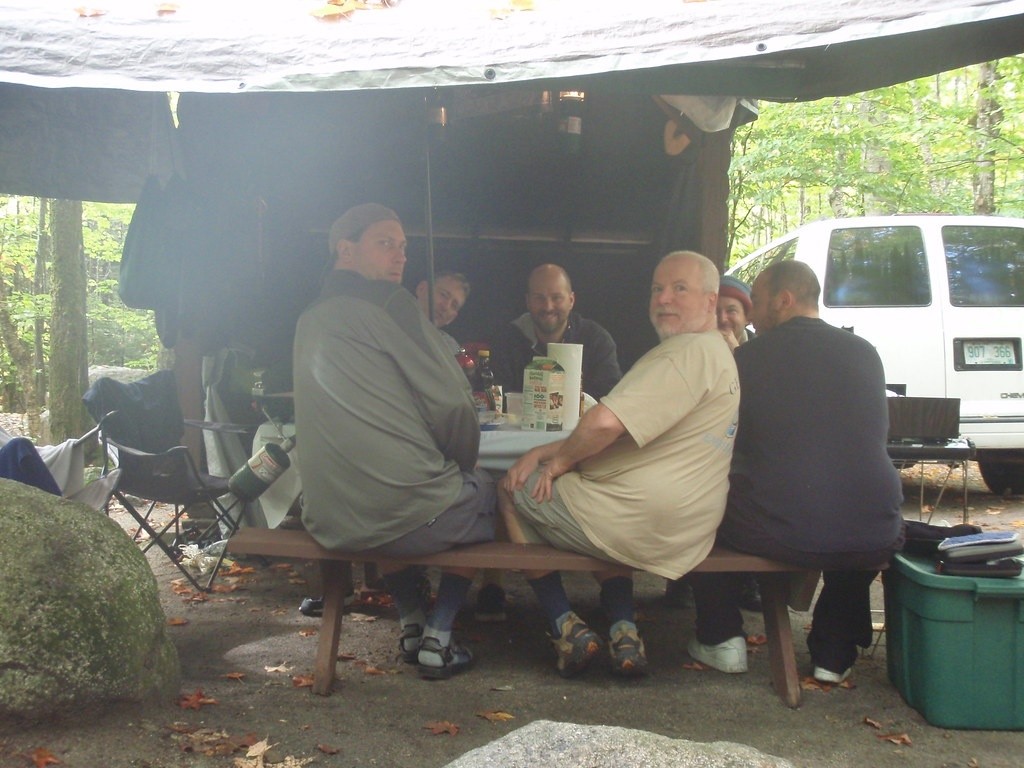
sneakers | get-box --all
[545,613,603,678]
[814,665,852,683]
[608,625,649,682]
[689,638,748,675]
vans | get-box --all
[714,213,1024,493]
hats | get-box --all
[325,204,401,270]
[719,275,752,315]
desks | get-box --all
[886,437,976,526]
[249,413,575,533]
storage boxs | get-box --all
[491,385,503,413]
[880,550,1024,732]
[520,356,567,432]
[505,392,523,428]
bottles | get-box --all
[579,370,585,425]
[228,438,296,503]
[472,351,494,412]
[455,348,476,385]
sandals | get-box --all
[417,638,472,681]
[398,625,425,664]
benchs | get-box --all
[229,527,824,712]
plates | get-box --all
[480,423,500,430]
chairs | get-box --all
[0,408,125,519]
[78,367,269,594]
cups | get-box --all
[504,392,523,424]
[493,386,502,413]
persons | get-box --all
[687,260,905,683]
[292,205,482,675]
[715,277,762,611]
[475,264,623,622]
[498,252,741,677]
[298,266,471,615]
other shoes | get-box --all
[666,575,695,609]
[298,586,354,615]
[418,574,433,611]
[740,580,761,611]
[477,584,505,621]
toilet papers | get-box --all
[546,342,584,431]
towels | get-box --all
[0,426,84,498]
